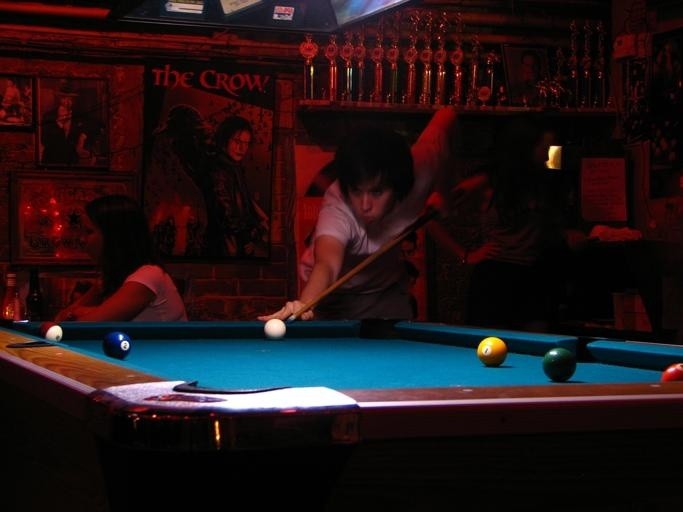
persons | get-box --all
[147,133,208,263]
[53,193,189,322]
[256,105,456,323]
[202,116,270,256]
[426,121,578,330]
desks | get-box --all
[0,316,683,512]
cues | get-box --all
[289,187,467,321]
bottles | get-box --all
[0,271,23,319]
[565,17,612,111]
[23,269,45,322]
[145,223,206,259]
[303,9,499,105]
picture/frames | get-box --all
[8,170,140,270]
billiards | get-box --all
[662,363,683,383]
[41,322,62,342]
[103,332,131,360]
[543,348,576,382]
[265,318,285,339]
[477,336,506,367]
